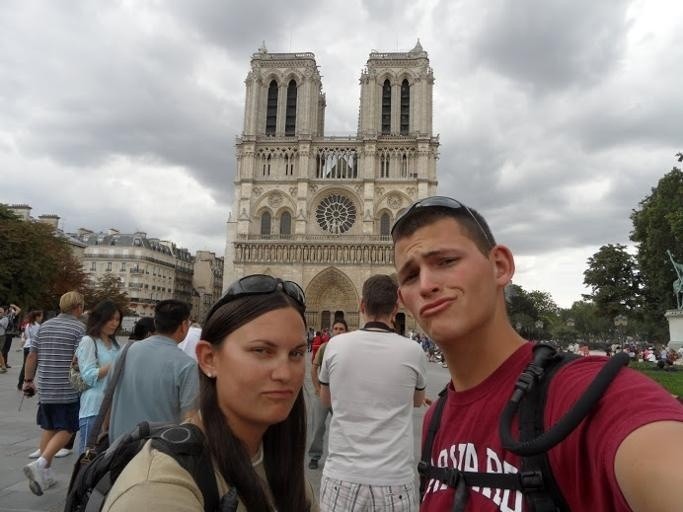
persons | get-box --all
[28,301,202,459]
[391,196,682,511]
[100,271,325,511]
[408,328,446,370]
[316,273,432,511]
[306,326,330,363]
[22,293,86,497]
[0,303,43,393]
[306,317,349,470]
[566,335,682,367]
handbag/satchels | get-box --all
[89,432,109,453]
[68,365,101,392]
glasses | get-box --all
[391,196,492,250]
[204,275,307,323]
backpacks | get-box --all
[64,420,140,511]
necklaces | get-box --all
[198,409,264,469]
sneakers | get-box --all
[308,459,319,471]
[23,448,73,496]
